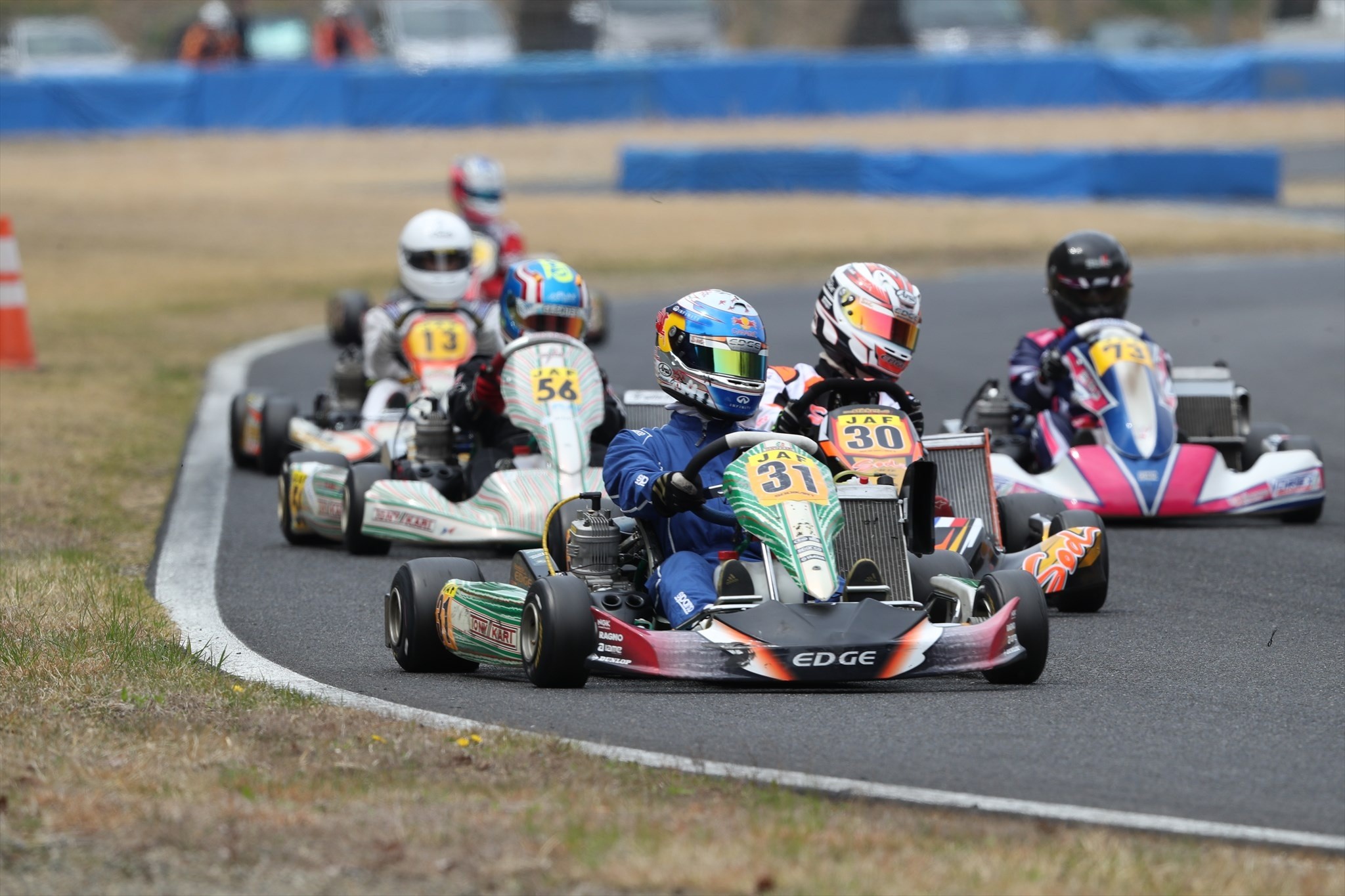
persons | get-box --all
[753,261,954,520]
[450,250,623,498]
[312,0,380,65]
[603,290,883,630]
[180,0,241,67]
[1011,227,1190,473]
[362,210,499,460]
[447,153,522,296]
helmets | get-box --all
[811,261,921,380]
[498,256,592,343]
[450,154,508,221]
[654,289,770,421]
[397,208,473,303]
[1047,230,1134,326]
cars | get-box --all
[846,1,1055,57]
[360,0,521,75]
[0,13,135,79]
[566,0,726,62]
[1076,17,1198,50]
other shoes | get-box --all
[1074,428,1096,447]
[843,558,887,601]
[469,446,507,489]
[717,559,755,613]
[1177,428,1188,442]
[934,496,955,517]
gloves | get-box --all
[472,364,505,412]
[1040,349,1072,396]
[652,472,707,519]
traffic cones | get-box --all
[0,214,47,373]
